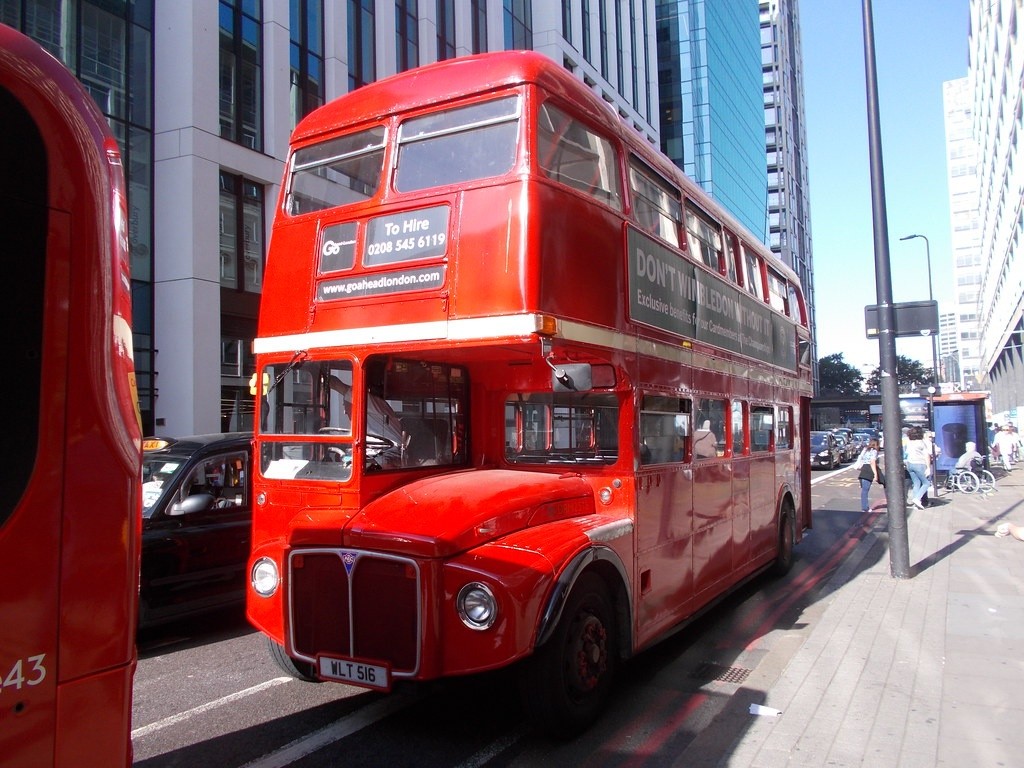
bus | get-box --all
[0,22,143,768]
[247,51,815,745]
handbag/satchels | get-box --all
[853,458,864,470]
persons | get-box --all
[790,428,800,491]
[303,363,445,470]
[152,465,192,497]
[694,420,718,459]
[946,441,983,489]
[902,426,941,510]
[987,422,1023,472]
[859,438,886,512]
[506,428,536,458]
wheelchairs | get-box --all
[944,455,995,494]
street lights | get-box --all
[927,387,936,497]
[900,234,938,387]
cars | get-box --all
[810,431,841,471]
[852,433,871,454]
[826,428,856,463]
[857,427,884,451]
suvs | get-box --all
[139,432,314,630]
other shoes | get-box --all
[863,509,872,512]
[912,498,925,510]
[921,500,934,506]
